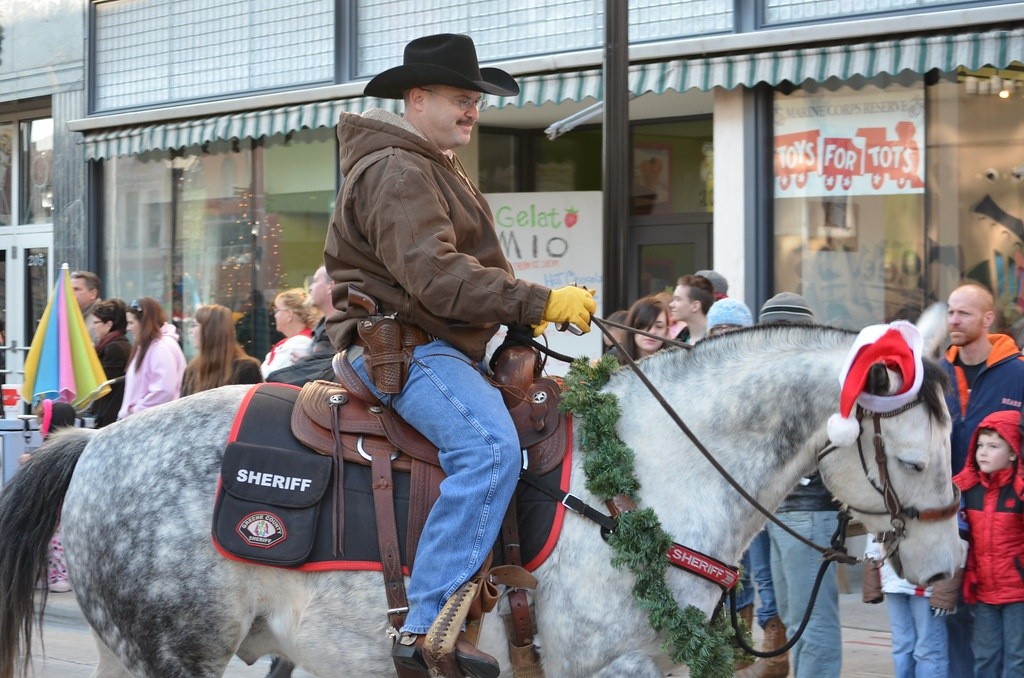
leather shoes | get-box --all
[394,630,500,678]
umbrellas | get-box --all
[20,262,113,414]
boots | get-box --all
[733,606,789,678]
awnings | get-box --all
[65,0,1024,161]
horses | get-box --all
[0,301,968,677]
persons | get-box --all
[863,267,1024,678]
[69,266,340,678]
[600,269,789,678]
[179,306,264,400]
[758,292,842,678]
[951,410,1024,678]
[17,403,85,593]
[320,34,596,678]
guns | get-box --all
[346,283,404,395]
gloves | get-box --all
[531,286,597,338]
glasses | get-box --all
[130,299,144,314]
[419,88,487,111]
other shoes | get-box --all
[34,577,73,593]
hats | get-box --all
[364,33,519,97]
[706,297,754,335]
[758,291,815,323]
[693,270,728,295]
